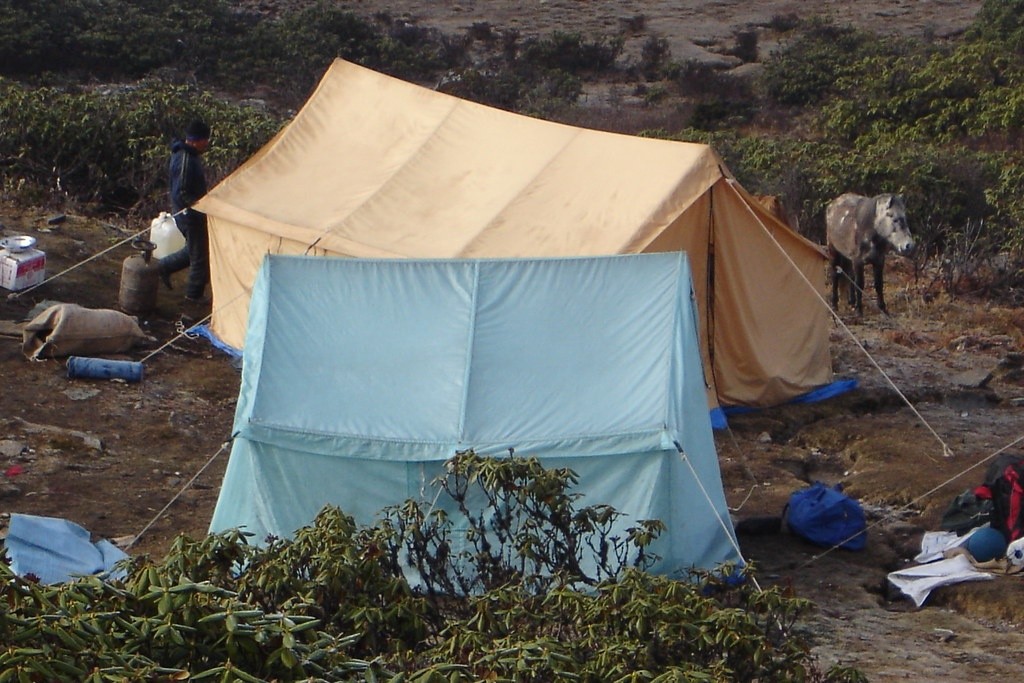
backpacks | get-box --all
[782,480,866,551]
[941,453,1024,545]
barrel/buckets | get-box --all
[150,212,185,260]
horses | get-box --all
[824,188,915,320]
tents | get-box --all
[190,56,836,434]
[201,248,744,607]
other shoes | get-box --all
[184,295,210,305]
[162,278,173,291]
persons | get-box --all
[156,118,215,306]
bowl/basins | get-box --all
[0,236,36,252]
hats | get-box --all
[186,119,211,140]
